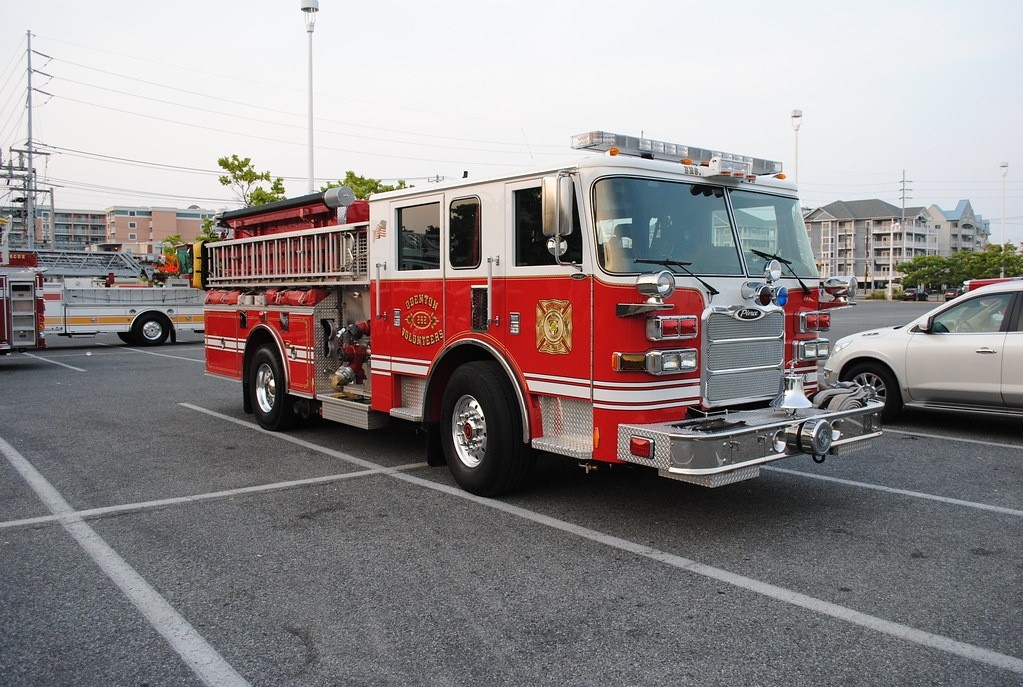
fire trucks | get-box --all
[202,131,887,499]
[0,214,207,355]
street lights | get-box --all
[300,0,319,196]
[791,109,802,183]
[1000,161,1009,278]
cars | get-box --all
[823,278,1023,425]
[901,287,918,302]
[918,289,928,301]
[945,288,962,301]
